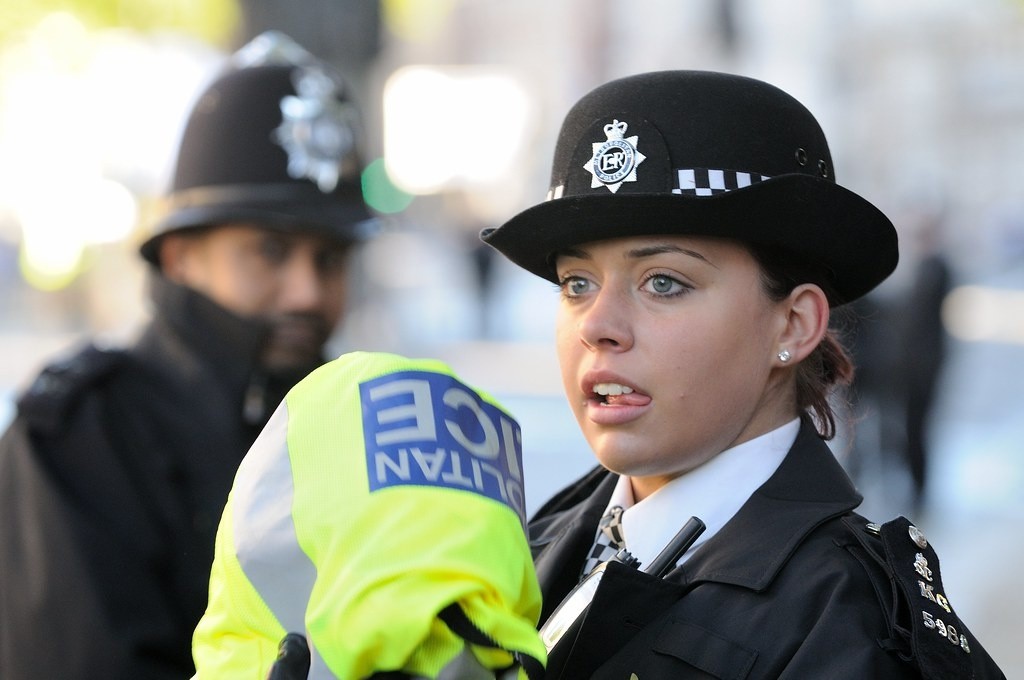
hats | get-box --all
[139,60,375,259]
[480,70,900,309]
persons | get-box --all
[479,69,1006,679]
[0,63,378,680]
